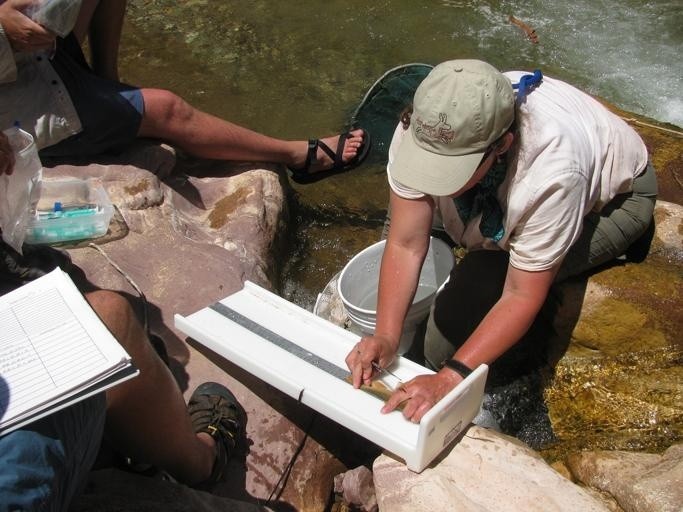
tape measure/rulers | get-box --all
[345,372,409,412]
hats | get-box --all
[390,58,515,197]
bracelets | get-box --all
[443,359,472,379]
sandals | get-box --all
[179,382,239,490]
[293,130,370,184]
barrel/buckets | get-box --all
[337,236,456,364]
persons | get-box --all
[345,58,658,423]
[0,290,290,512]
[0,1,370,184]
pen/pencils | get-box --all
[358,351,402,381]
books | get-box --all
[0,266,142,435]
